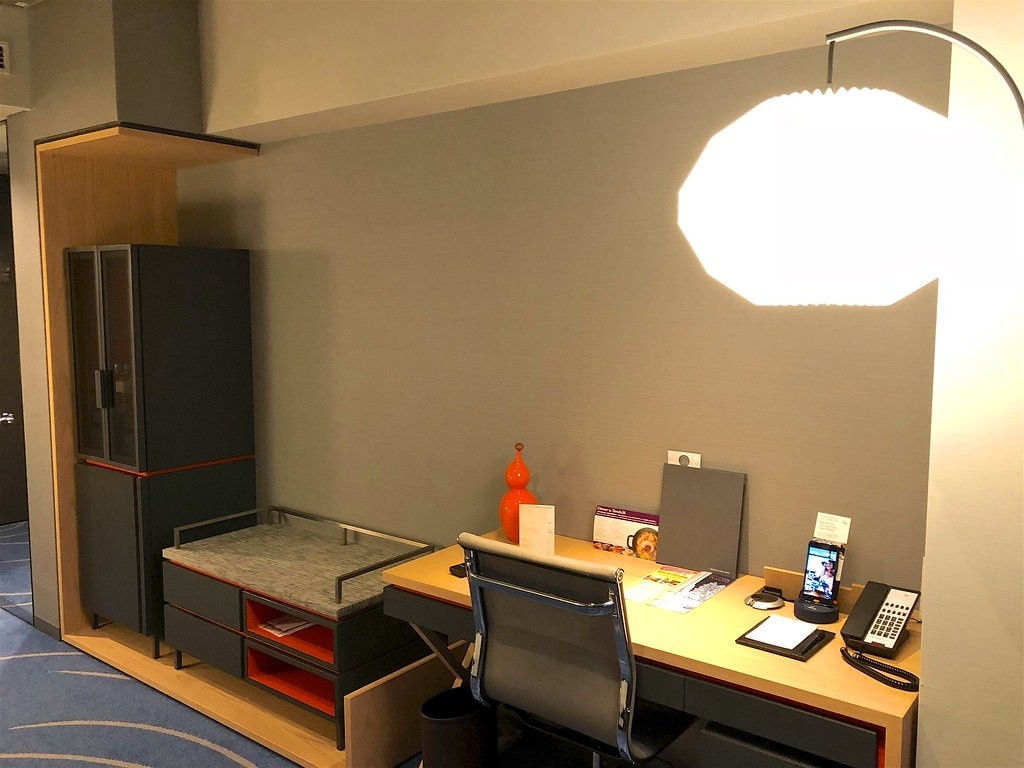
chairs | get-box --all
[456,531,702,768]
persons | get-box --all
[814,558,836,594]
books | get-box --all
[735,616,836,663]
[258,612,314,637]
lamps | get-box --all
[677,20,1024,307]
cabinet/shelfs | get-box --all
[162,504,449,750]
[62,244,257,660]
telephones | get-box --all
[839,580,921,660]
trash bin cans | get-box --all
[418,686,498,768]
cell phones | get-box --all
[801,541,840,609]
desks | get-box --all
[384,530,922,768]
